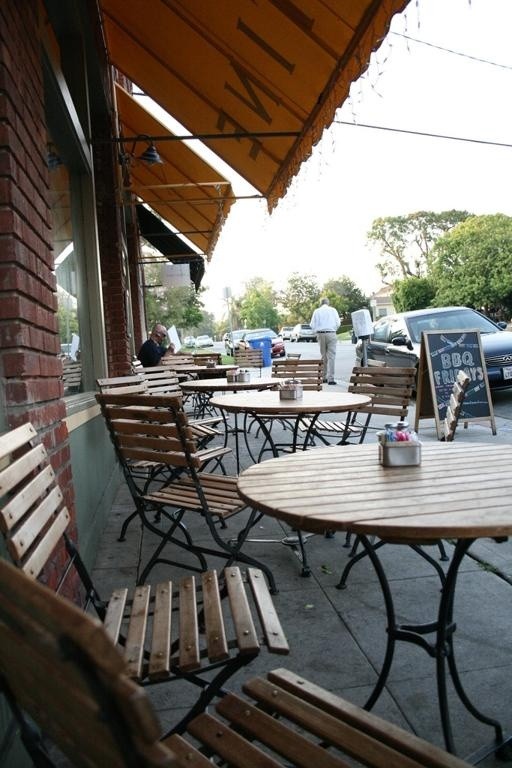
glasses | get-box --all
[162,333,166,337]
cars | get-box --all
[195,335,214,348]
[278,327,293,339]
[355,307,511,397]
[225,331,242,356]
[239,329,285,357]
[184,336,195,345]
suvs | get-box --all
[290,324,317,342]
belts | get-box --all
[317,330,336,334]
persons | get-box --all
[309,296,342,385]
[137,323,188,406]
[75,350,81,359]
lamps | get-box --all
[120,133,164,167]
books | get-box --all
[166,324,181,354]
[70,334,80,361]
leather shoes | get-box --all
[323,379,328,383]
[328,382,337,385]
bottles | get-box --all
[239,369,251,382]
[289,380,302,397]
[385,421,409,432]
